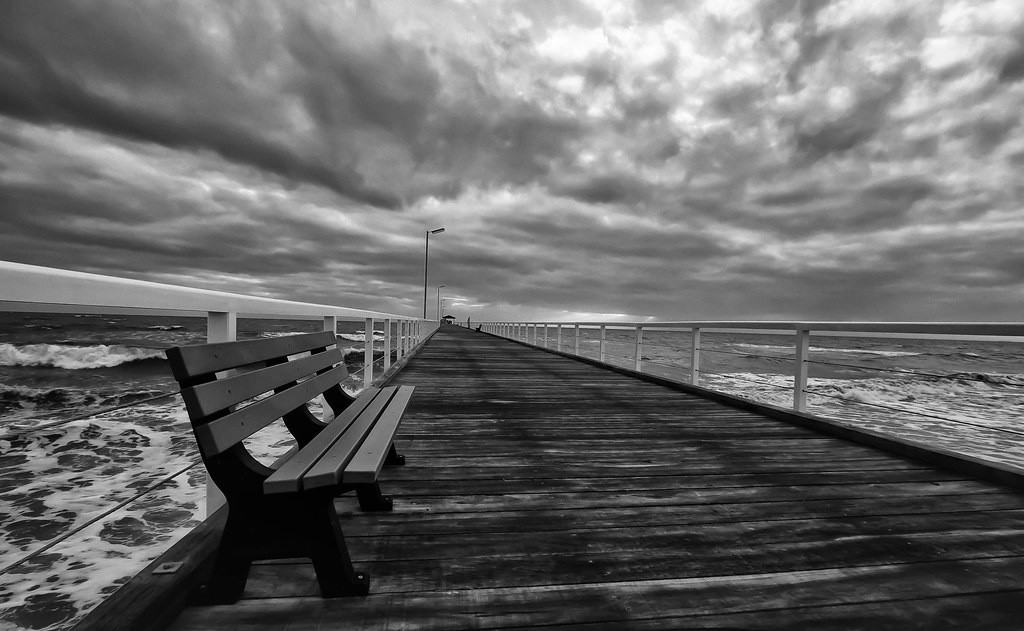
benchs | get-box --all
[165,331,416,606]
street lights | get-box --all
[424,227,446,320]
[440,299,446,318]
[437,285,445,320]
